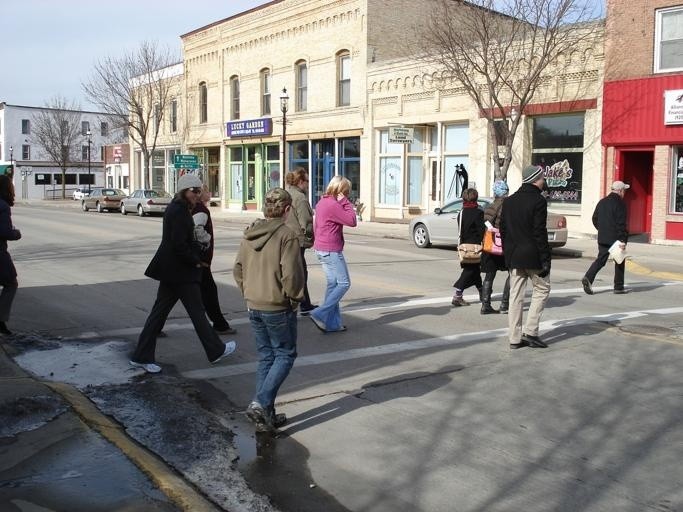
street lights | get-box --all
[9,145,14,164]
[87,128,93,191]
[277,87,292,189]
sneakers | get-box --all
[582,277,594,295]
[212,328,237,335]
[254,412,287,432]
[245,401,281,440]
[129,360,162,373]
[451,296,471,306]
[308,315,348,334]
[300,305,319,316]
[210,339,236,365]
[614,287,632,294]
[509,334,547,350]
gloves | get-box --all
[537,260,551,278]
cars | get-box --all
[81,188,125,213]
[408,196,568,251]
[71,187,89,200]
[118,189,173,216]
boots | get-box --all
[500,279,510,313]
[479,280,500,314]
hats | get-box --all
[611,181,631,191]
[492,180,510,198]
[521,165,545,184]
[178,174,203,193]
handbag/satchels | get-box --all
[456,244,482,264]
[482,219,504,257]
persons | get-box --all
[0,172,21,337]
[283,167,318,315]
[498,164,551,349]
[233,187,305,432]
[477,180,512,315]
[451,187,484,307]
[128,174,236,375]
[153,182,237,338]
[306,174,356,333]
[579,179,631,295]
